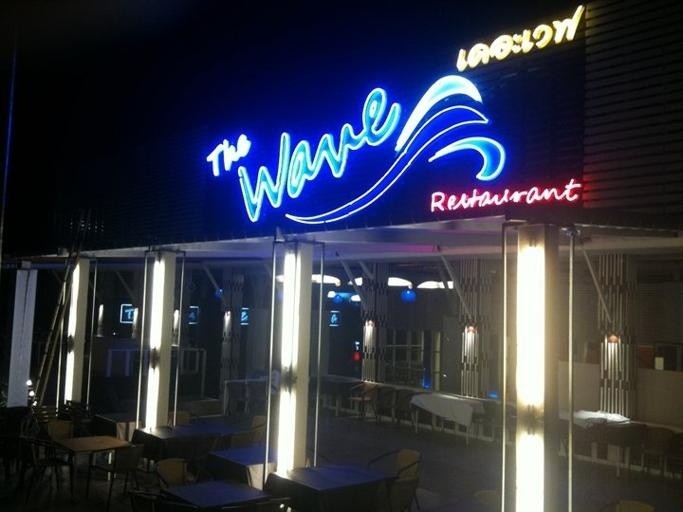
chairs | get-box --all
[310,378,681,479]
[0,396,424,511]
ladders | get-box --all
[15,245,82,485]
